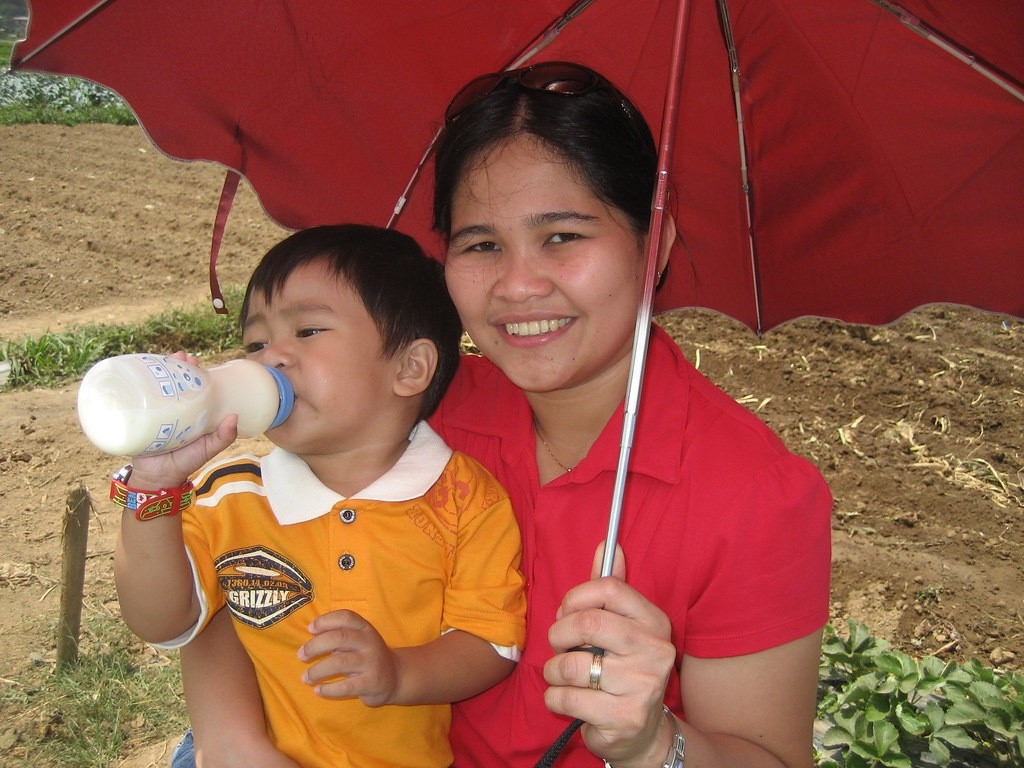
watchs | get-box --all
[602,703,686,768]
[110,463,195,521]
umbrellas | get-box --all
[0,0,1024,341]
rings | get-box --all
[589,653,603,690]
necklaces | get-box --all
[533,418,572,472]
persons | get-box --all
[114,223,526,768]
[171,65,833,768]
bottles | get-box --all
[77,353,295,457]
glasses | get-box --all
[444,60,649,157]
